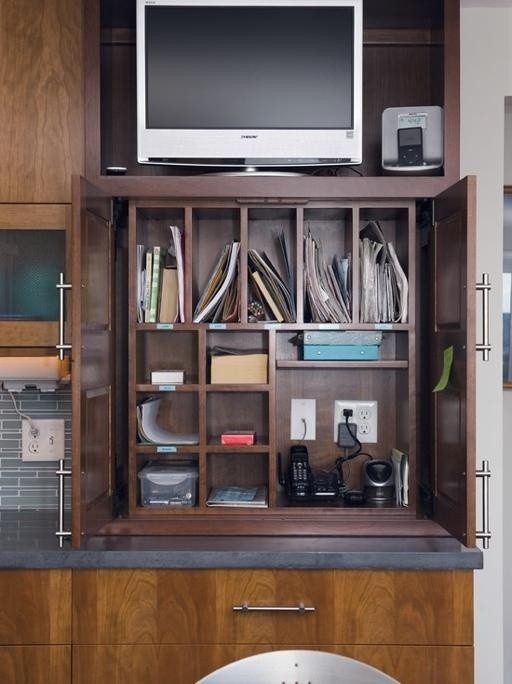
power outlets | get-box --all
[333,400,377,445]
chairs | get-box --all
[193,650,399,684]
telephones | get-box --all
[285,444,340,503]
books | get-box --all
[304,331,383,361]
[252,271,287,324]
[192,240,242,323]
[388,241,408,323]
[136,225,185,324]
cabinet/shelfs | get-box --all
[69,569,473,684]
[0,569,71,683]
[0,0,100,230]
[56,173,493,549]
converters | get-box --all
[338,423,357,447]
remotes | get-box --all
[105,166,126,176]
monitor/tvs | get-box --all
[136,0,364,179]
